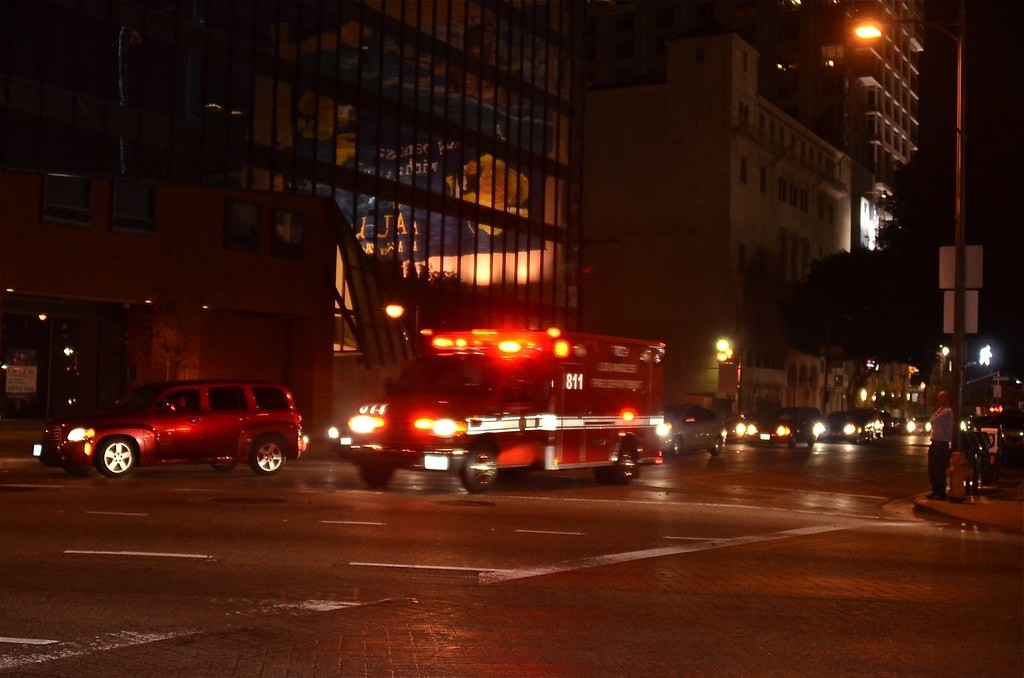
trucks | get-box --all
[345,324,667,499]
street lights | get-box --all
[847,14,965,447]
[381,297,422,358]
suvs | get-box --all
[41,379,302,480]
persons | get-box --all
[928,390,954,500]
[172,395,190,414]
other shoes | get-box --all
[927,493,945,500]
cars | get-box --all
[721,412,759,442]
[650,402,727,459]
[880,401,1024,452]
[754,406,823,445]
[816,405,889,446]
